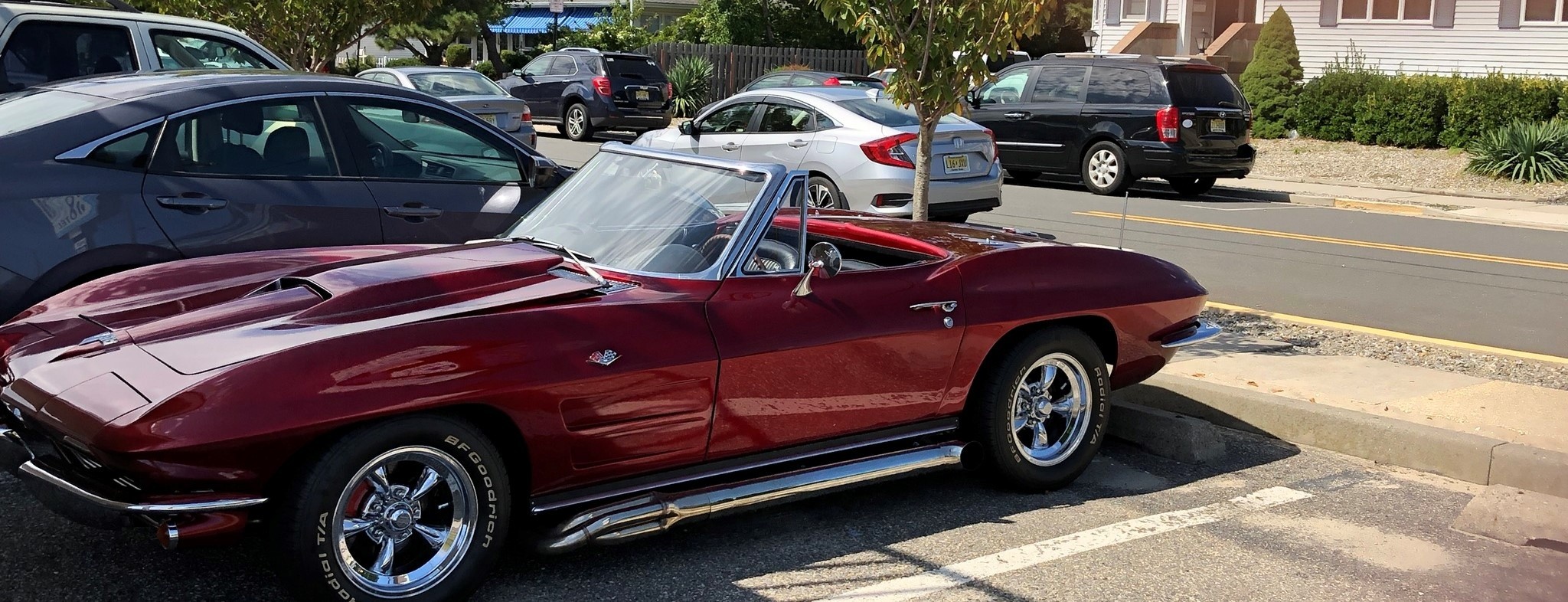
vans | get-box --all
[955,53,1257,195]
[0,3,326,163]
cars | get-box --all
[856,68,921,87]
[693,71,896,119]
[629,85,1005,223]
[935,50,1033,104]
[349,66,537,152]
[0,68,588,319]
[0,141,1224,602]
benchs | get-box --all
[717,222,901,274]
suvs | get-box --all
[492,47,672,141]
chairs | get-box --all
[93,54,122,73]
[264,127,313,173]
[771,108,796,131]
[220,106,265,175]
[748,237,799,272]
